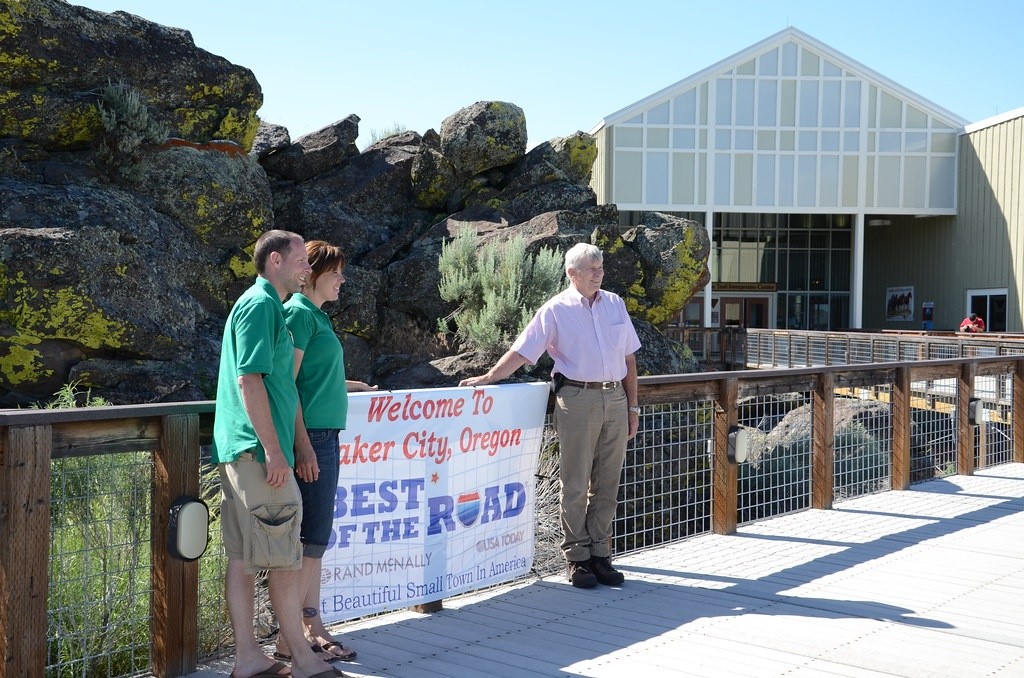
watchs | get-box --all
[628,407,642,415]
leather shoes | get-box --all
[567,555,624,589]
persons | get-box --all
[209,229,351,678]
[960,313,985,357]
[458,243,641,590]
[274,241,379,662]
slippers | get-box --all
[229,640,357,677]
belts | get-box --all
[561,376,623,390]
